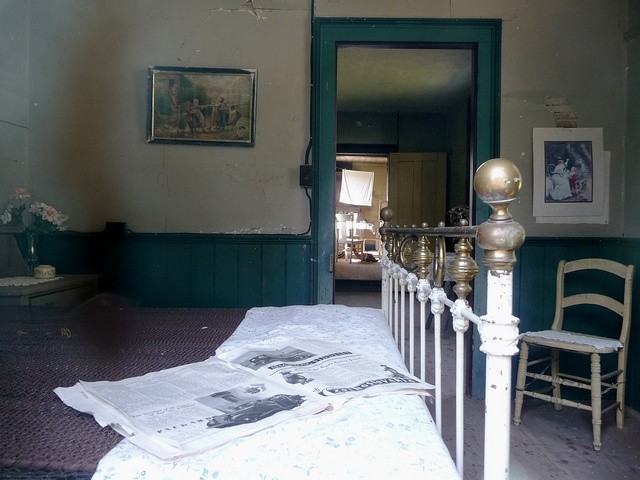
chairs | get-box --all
[514,258,635,453]
[335,213,366,265]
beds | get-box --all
[0,158,549,480]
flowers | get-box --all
[1,186,69,232]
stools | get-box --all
[426,252,457,340]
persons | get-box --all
[174,97,242,135]
[282,369,314,385]
[551,156,573,201]
[567,166,578,194]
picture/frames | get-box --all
[145,66,258,147]
[532,127,605,218]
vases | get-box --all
[22,234,40,276]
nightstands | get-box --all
[1,271,96,307]
